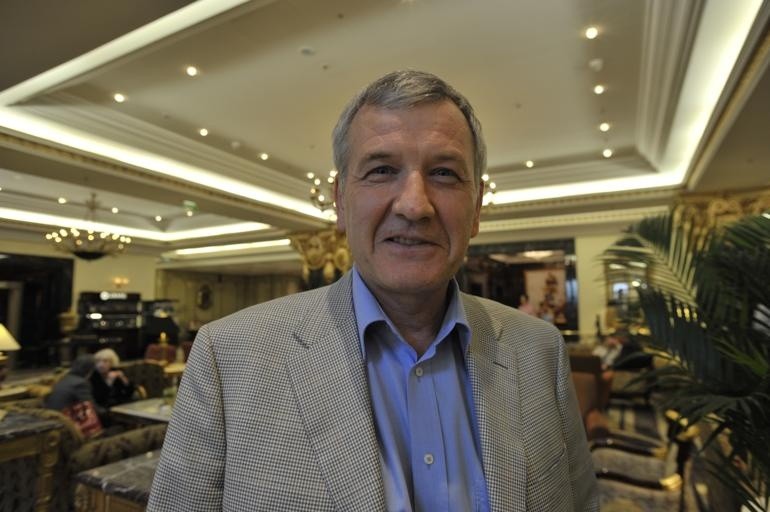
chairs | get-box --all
[583,403,742,511]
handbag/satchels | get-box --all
[61,402,103,440]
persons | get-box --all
[89,346,136,429]
[141,66,606,512]
[46,353,107,418]
[537,301,556,324]
[515,292,536,316]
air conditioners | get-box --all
[43,190,132,261]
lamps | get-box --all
[284,175,355,284]
[1,324,22,380]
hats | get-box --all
[73,355,96,371]
